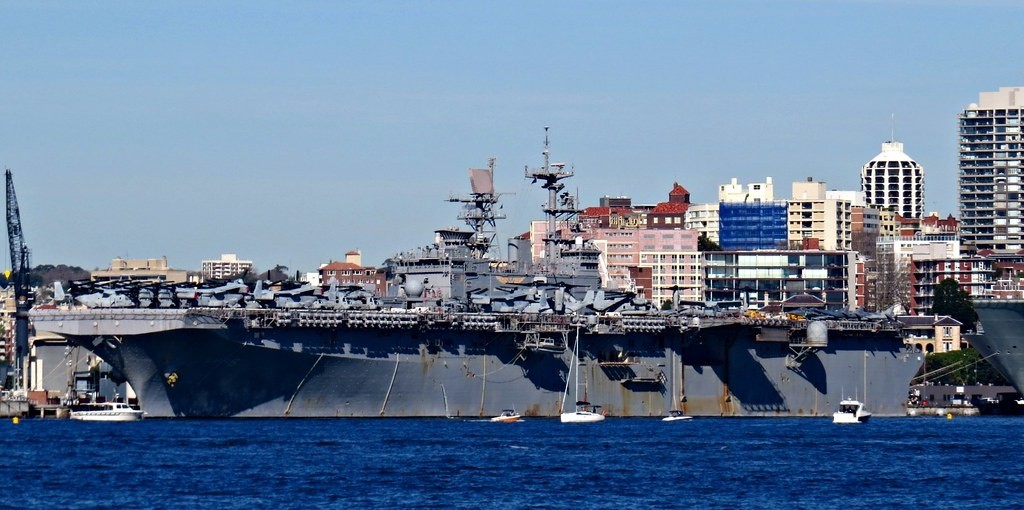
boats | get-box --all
[961,271,1024,401]
[490,403,522,423]
[832,385,872,423]
[68,386,146,421]
[560,401,606,423]
[662,409,693,422]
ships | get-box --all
[0,124,925,419]
